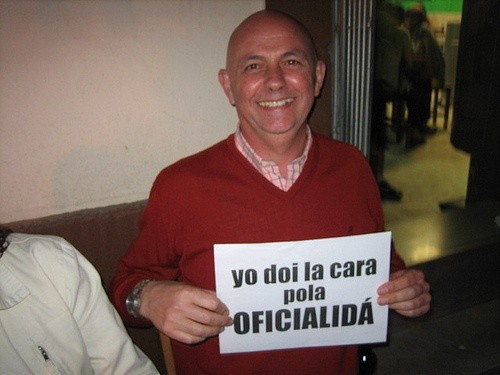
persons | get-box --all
[107,9,432,375]
[0,225,160,375]
[370,4,445,202]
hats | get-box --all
[407,3,426,17]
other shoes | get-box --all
[439,201,495,224]
[378,181,401,200]
[405,136,425,146]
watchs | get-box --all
[125,279,150,319]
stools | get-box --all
[383,95,408,143]
[428,85,452,130]
